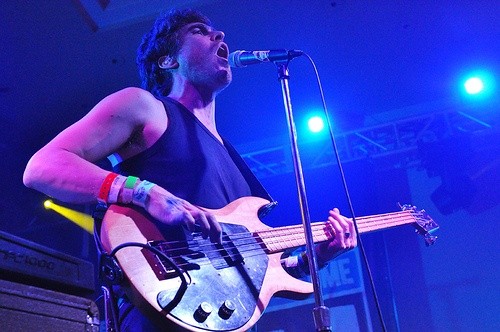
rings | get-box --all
[344,232,351,238]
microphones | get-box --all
[228,50,304,69]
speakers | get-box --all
[0,280,100,332]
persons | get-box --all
[23,9,356,332]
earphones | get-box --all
[164,55,172,64]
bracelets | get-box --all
[96,172,156,208]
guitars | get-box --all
[99,196,439,332]
